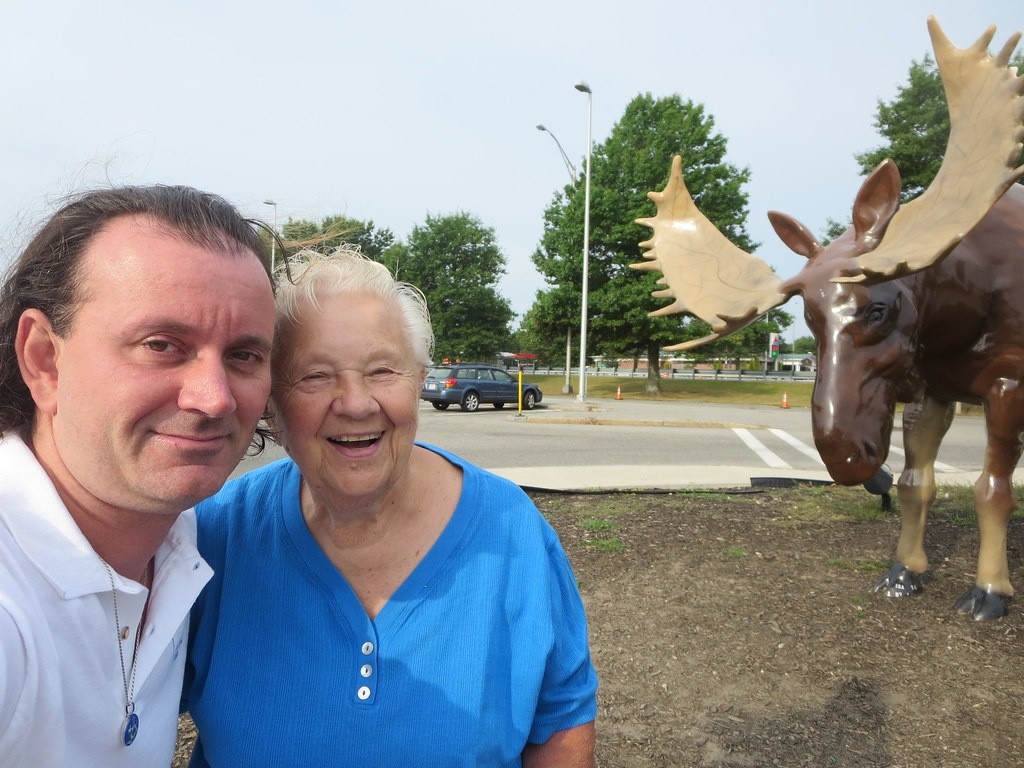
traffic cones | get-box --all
[615,386,623,400]
[780,392,790,409]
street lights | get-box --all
[536,124,578,395]
[264,199,277,272]
[575,83,592,401]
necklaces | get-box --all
[97,553,148,746]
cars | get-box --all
[421,365,542,412]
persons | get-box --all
[0,184,317,768]
[179,241,598,768]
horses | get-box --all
[767,159,1024,625]
